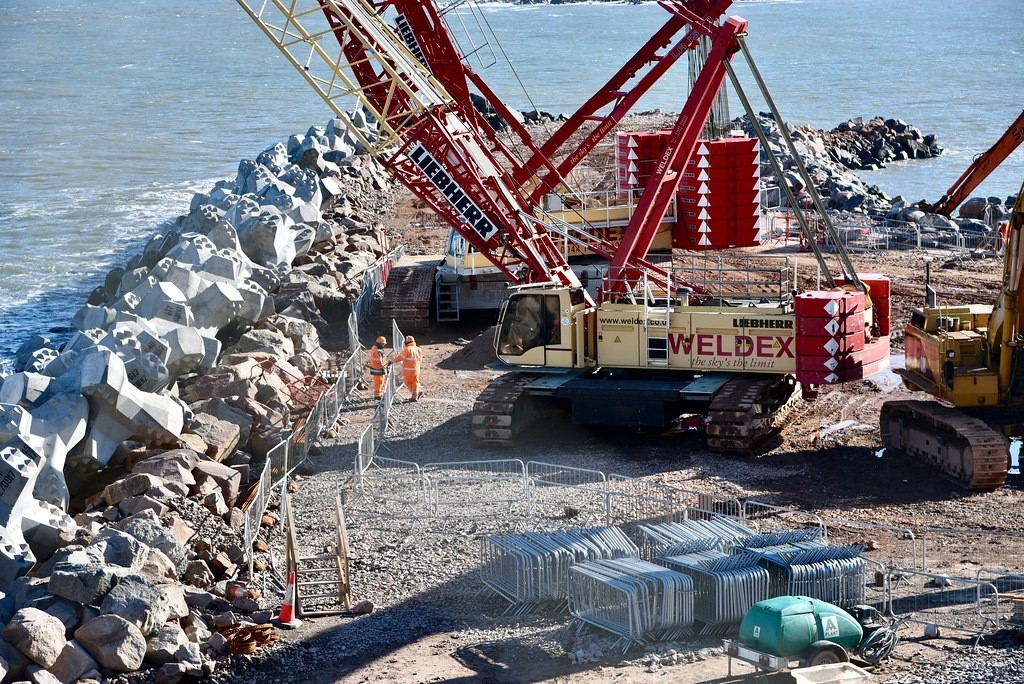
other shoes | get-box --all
[418,392,423,400]
[375,396,381,400]
[409,398,417,402]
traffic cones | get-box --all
[225,580,251,602]
[270,571,302,629]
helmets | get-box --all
[405,336,415,342]
[376,336,387,344]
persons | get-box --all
[370,336,386,399]
[388,336,422,401]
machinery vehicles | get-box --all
[878,110,1024,493]
[234,0,893,459]
